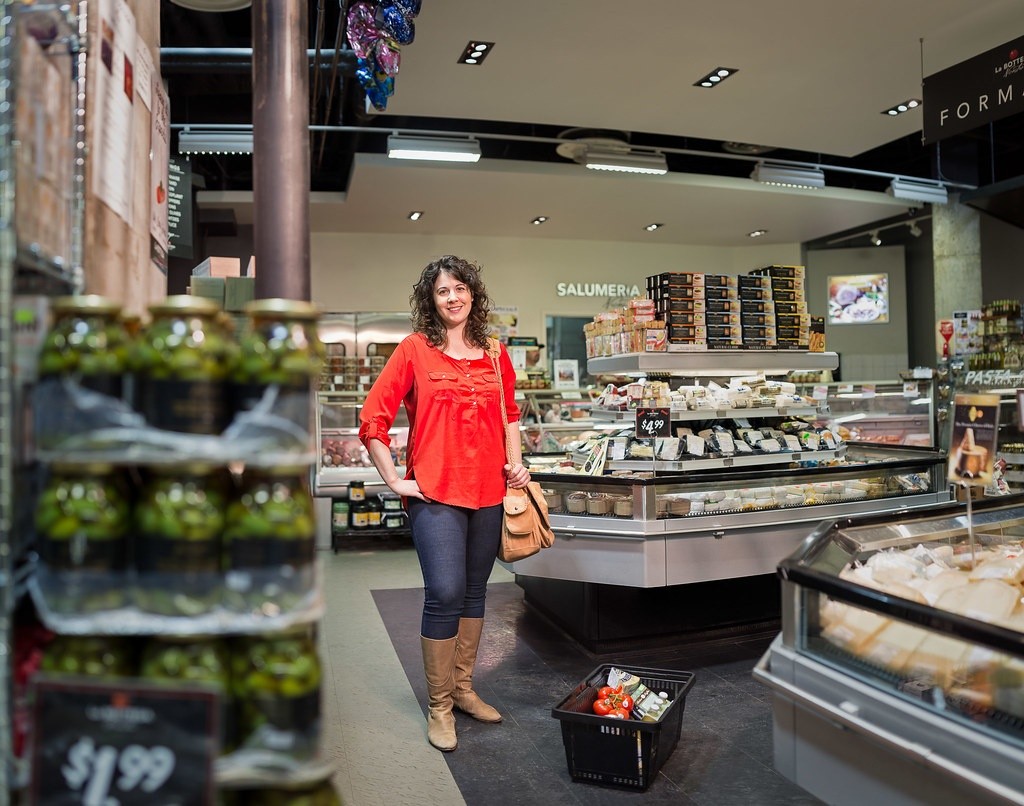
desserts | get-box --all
[954,428,988,478]
[540,489,691,515]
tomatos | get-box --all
[593,686,634,720]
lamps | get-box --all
[179,131,253,153]
[752,161,825,189]
[387,135,482,163]
[910,223,922,237]
[871,232,881,246]
[886,179,948,204]
[583,145,668,175]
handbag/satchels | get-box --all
[498,480,555,562]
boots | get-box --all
[451,618,502,723]
[419,634,458,751]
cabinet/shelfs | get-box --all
[1,2,94,804]
[573,351,848,473]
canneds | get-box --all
[21,292,339,806]
[332,481,380,531]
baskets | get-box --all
[551,663,697,793]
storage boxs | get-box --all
[644,265,825,353]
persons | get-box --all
[358,254,532,752]
